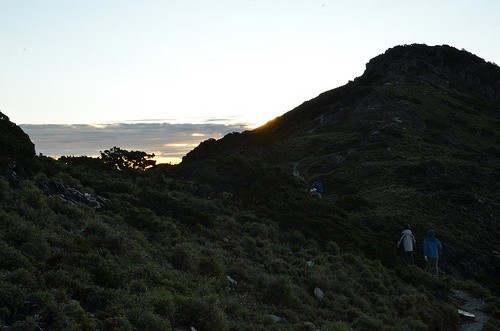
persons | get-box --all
[310,177,323,193]
[421,228,443,275]
[395,223,418,265]
